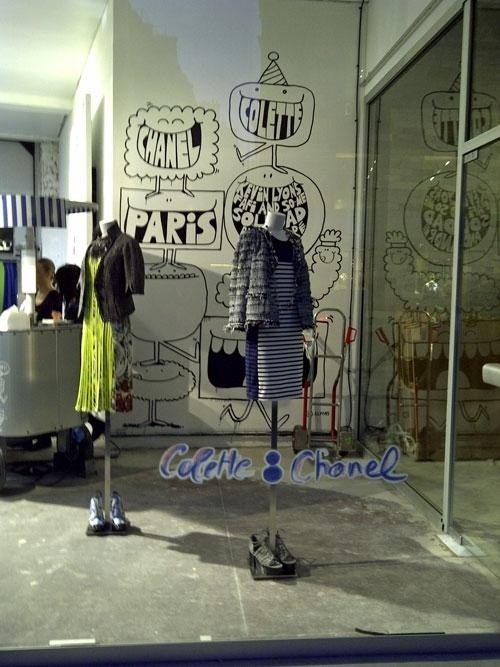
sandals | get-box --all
[248,528,296,569]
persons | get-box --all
[228,212,313,402]
[75,222,144,413]
[35,259,83,325]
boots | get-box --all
[111,490,128,531]
[88,490,105,532]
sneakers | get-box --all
[91,418,105,441]
[34,437,51,450]
